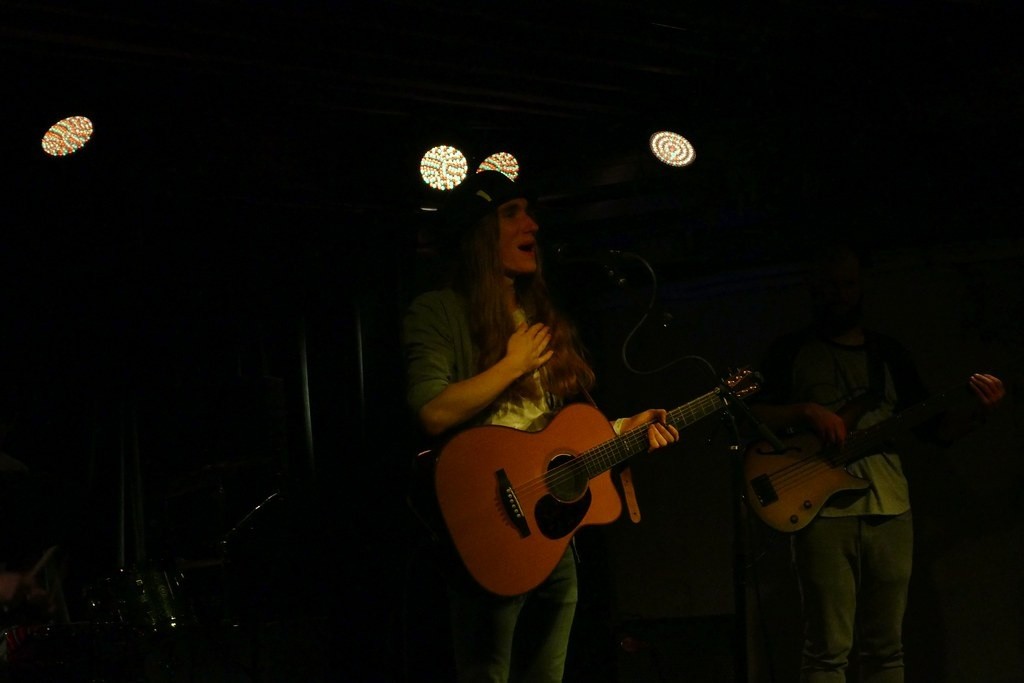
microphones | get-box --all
[551,239,622,261]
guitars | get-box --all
[405,365,759,598]
[734,381,975,534]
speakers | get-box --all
[587,612,735,683]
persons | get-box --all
[746,241,1018,681]
[0,551,30,604]
[399,166,683,683]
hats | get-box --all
[449,170,528,213]
